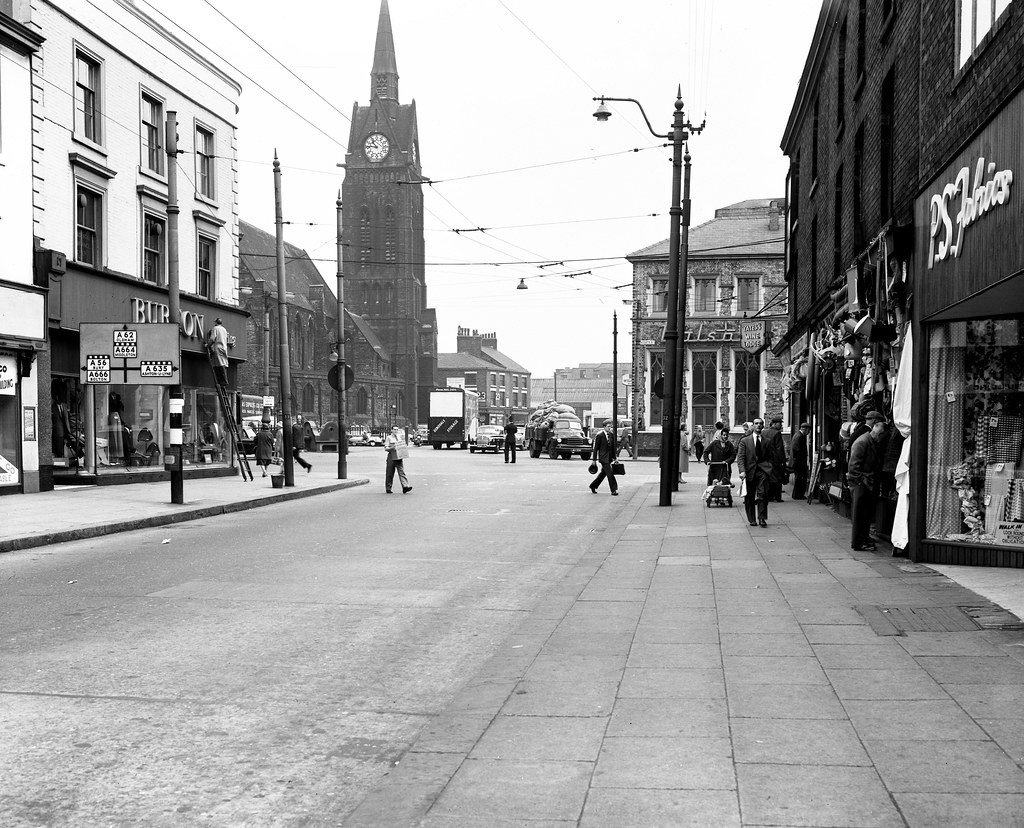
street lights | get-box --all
[589,82,708,508]
[327,341,348,479]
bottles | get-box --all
[825,442,836,452]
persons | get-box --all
[504,416,517,463]
[589,419,619,496]
[255,419,313,477]
[678,417,811,528]
[205,318,229,386]
[52,394,71,458]
[845,410,890,552]
[384,425,413,494]
[109,391,124,458]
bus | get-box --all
[428,384,481,450]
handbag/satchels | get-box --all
[611,461,625,475]
[782,469,790,484]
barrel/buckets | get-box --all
[271,475,285,488]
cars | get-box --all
[469,425,507,454]
[410,424,429,447]
[348,433,383,447]
[512,427,530,452]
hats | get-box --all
[262,423,269,429]
[588,464,598,474]
[801,422,812,430]
[866,410,883,419]
[277,421,284,428]
[214,318,223,325]
[772,417,783,422]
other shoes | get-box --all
[759,518,767,526]
[589,484,597,493]
[750,521,757,525]
[386,490,393,493]
[611,491,619,495]
[403,486,412,493]
[679,479,688,483]
[853,541,875,550]
[510,461,516,463]
[505,460,509,463]
[307,464,312,473]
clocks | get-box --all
[361,131,391,163]
[411,141,417,169]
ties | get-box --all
[756,435,762,458]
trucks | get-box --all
[524,418,594,460]
